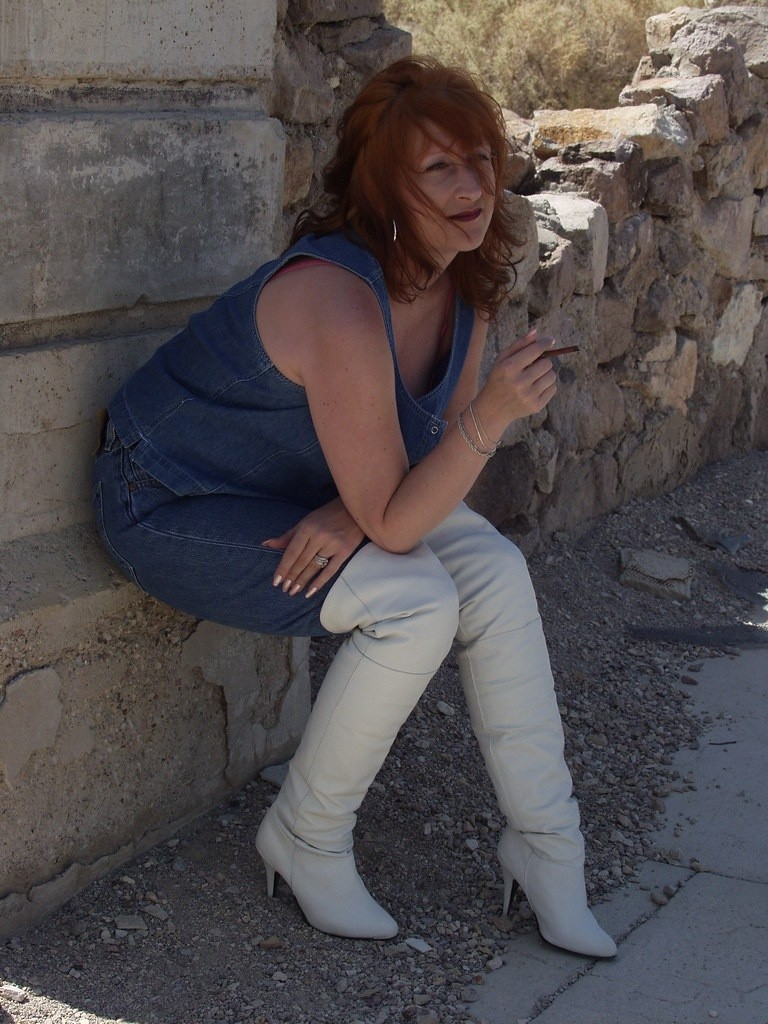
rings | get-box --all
[315,554,328,567]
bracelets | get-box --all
[458,398,502,457]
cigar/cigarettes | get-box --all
[540,345,582,358]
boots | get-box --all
[425,499,620,962]
[257,541,460,940]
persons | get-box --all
[89,61,617,958]
